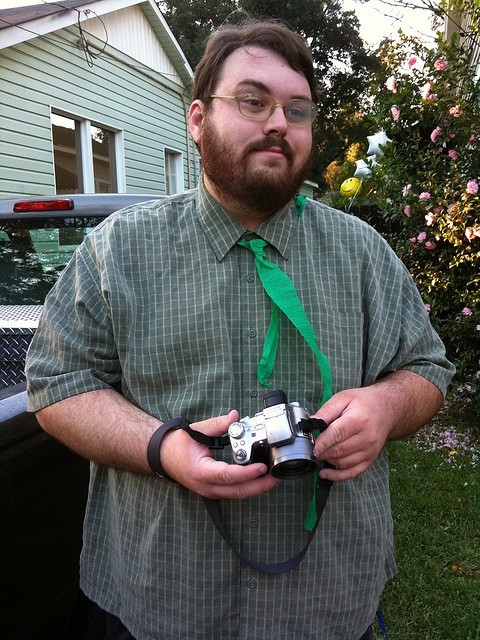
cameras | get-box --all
[228,389,316,482]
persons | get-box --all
[24,8,456,640]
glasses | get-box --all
[210,92,321,128]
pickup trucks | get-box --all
[0,193,160,639]
